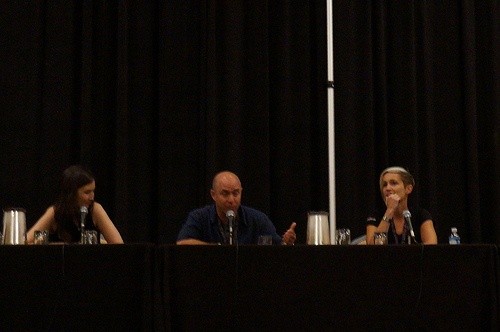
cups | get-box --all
[336,229,351,245]
[83,231,98,244]
[258,234,272,244]
[34,230,49,244]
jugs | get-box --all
[307,212,330,245]
[1,209,26,244]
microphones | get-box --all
[80,206,88,228]
[403,210,415,238]
[225,210,236,232]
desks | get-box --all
[0,241,500,332]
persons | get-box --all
[366,167,436,244]
[176,171,296,244]
[26,171,124,244]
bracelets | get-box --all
[382,216,391,222]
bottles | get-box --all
[449,228,461,244]
